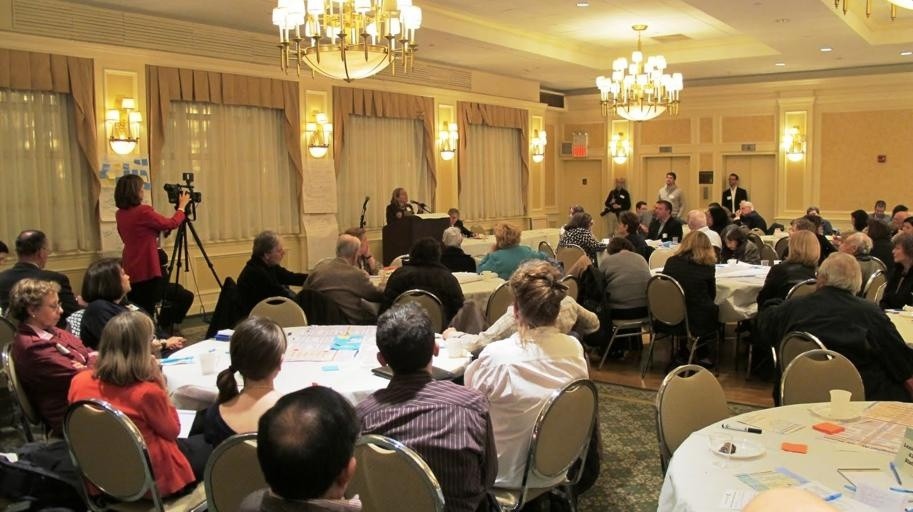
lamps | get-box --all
[609,132,632,164]
[272,0,422,83]
[835,0,913,22]
[104,97,143,154]
[435,121,458,160]
[571,132,589,158]
[781,125,807,161]
[595,25,684,122]
[532,129,547,163]
[306,110,333,159]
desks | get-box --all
[884,309,913,349]
[657,400,913,512]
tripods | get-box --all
[167,206,223,324]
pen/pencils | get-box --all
[722,423,762,434]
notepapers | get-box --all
[813,423,845,435]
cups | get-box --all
[773,260,782,266]
[198,352,217,375]
[601,239,609,244]
[727,259,736,268]
[829,389,851,416]
[445,337,464,359]
[479,271,493,282]
[832,230,837,240]
[672,237,678,244]
[760,261,769,267]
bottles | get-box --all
[662,234,667,242]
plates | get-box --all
[810,405,861,421]
[708,438,766,459]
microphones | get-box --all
[363,196,370,210]
[410,200,431,213]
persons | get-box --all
[1,168,913,509]
